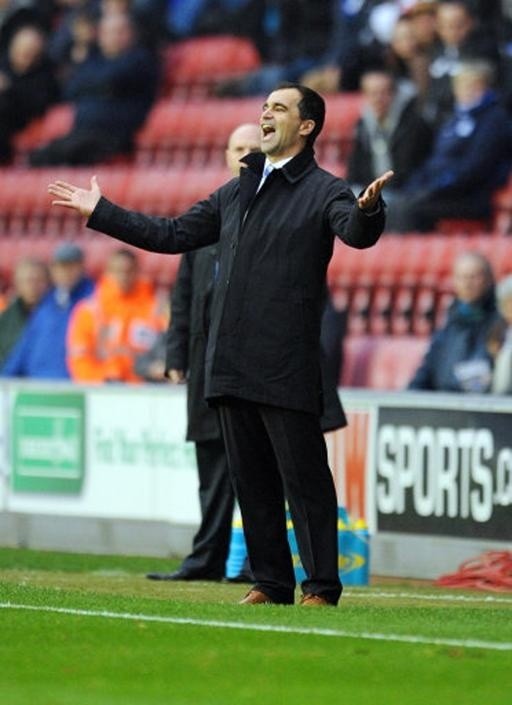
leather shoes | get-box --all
[301,593,328,605]
[225,574,255,583]
[147,571,222,580]
[240,590,270,604]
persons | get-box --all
[162,2,414,93]
[320,302,343,386]
[347,2,510,234]
[3,244,97,380]
[133,283,172,383]
[44,82,394,607]
[491,275,509,395]
[2,252,52,370]
[67,248,157,384]
[143,122,263,585]
[2,2,160,170]
[407,252,505,395]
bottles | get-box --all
[336,517,370,586]
